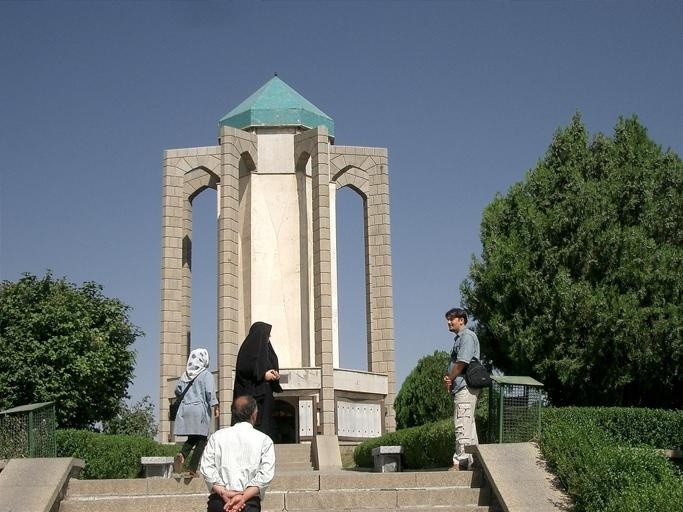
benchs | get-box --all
[140,455,174,479]
[372,445,404,472]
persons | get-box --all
[444,306,482,471]
[171,348,220,481]
[197,395,276,512]
[229,321,282,445]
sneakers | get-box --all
[448,463,466,471]
[175,452,196,475]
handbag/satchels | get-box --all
[464,361,491,388]
[168,396,183,421]
